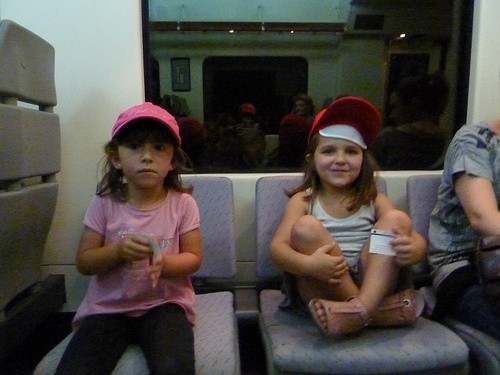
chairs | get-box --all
[1,18,62,358]
[30,175,241,375]
[254,177,469,375]
[405,173,500,374]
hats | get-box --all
[240,103,257,114]
[308,96,381,150]
[111,102,182,145]
[178,116,209,150]
[279,114,312,148]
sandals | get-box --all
[308,295,372,339]
[376,288,424,327]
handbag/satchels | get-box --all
[477,233,500,285]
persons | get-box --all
[221,101,266,165]
[375,84,448,171]
[271,94,314,171]
[427,119,500,340]
[55,102,203,375]
[270,97,428,338]
